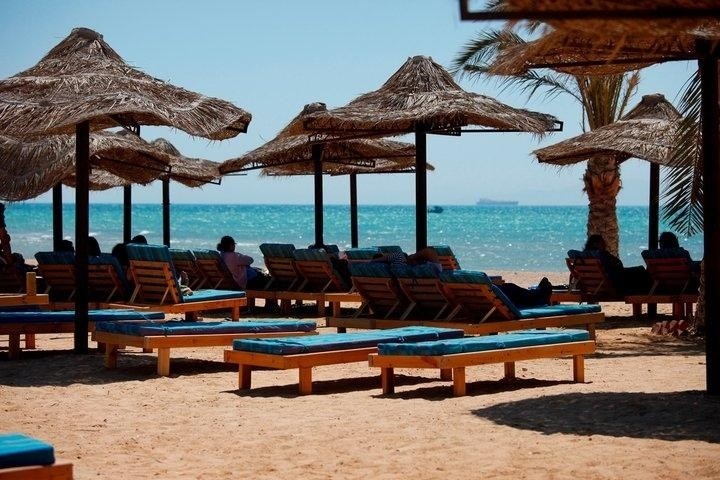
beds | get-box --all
[368,328,596,396]
[224,325,465,396]
[2,270,51,349]
[1,308,166,360]
[92,318,321,376]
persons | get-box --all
[11,233,189,303]
[658,232,702,294]
[407,253,552,309]
[581,235,665,320]
[217,236,281,317]
[313,243,353,293]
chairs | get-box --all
[192,245,605,346]
[1,244,248,322]
[563,248,702,322]
